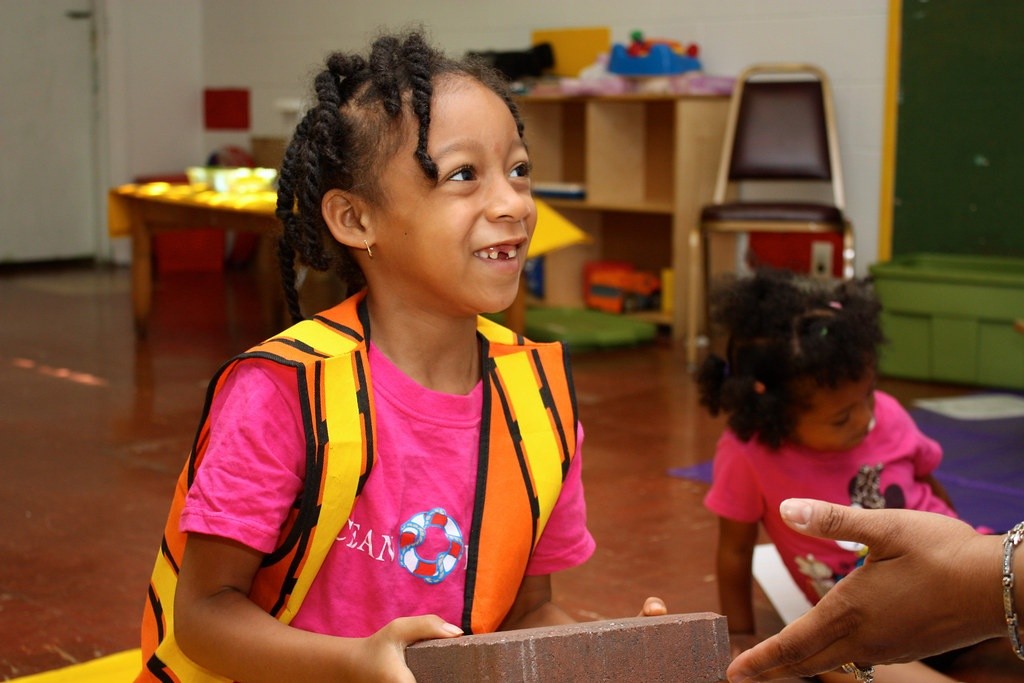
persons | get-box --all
[688,265,1023,683]
[723,491,1023,683]
[132,33,669,683]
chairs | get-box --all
[689,58,856,372]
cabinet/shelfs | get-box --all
[496,82,730,359]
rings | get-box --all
[842,661,876,683]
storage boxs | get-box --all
[868,251,1024,389]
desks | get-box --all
[106,183,596,343]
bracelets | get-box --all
[1000,521,1023,664]
